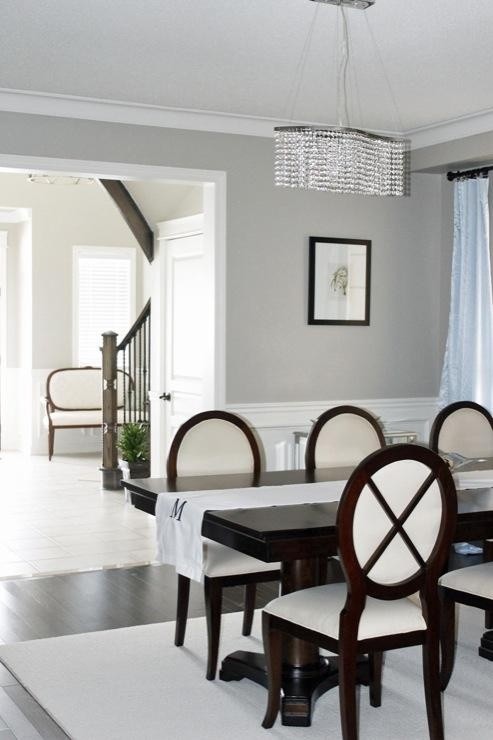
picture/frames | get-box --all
[306,234,375,327]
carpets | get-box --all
[1,601,492,740]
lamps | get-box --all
[268,2,411,199]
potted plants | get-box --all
[109,418,149,480]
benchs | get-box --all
[43,364,147,461]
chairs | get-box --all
[256,441,461,740]
[161,410,297,682]
[426,397,493,570]
[302,402,387,476]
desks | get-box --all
[108,454,493,729]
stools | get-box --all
[431,556,491,696]
